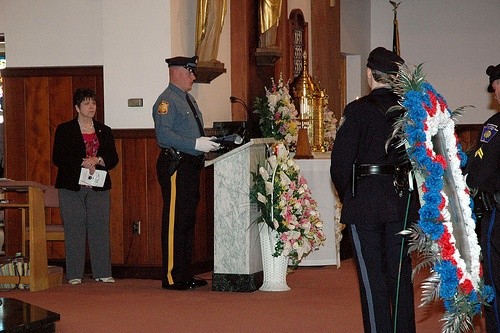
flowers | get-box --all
[385,59,495,333]
[253,74,345,272]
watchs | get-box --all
[97,156,102,164]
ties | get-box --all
[186,94,207,137]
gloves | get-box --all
[195,136,220,153]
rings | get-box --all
[90,164,92,165]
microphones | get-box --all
[230,96,263,138]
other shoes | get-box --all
[166,277,208,290]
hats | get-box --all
[486,64,500,93]
[165,55,197,69]
[367,47,405,73]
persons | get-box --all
[152,56,221,291]
[329,46,423,333]
[459,63,500,333]
[53,87,120,284]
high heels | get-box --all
[95,277,115,282]
[69,279,81,284]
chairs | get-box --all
[19,185,65,269]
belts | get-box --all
[355,164,409,175]
[163,151,194,160]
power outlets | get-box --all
[133,221,140,234]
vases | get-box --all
[256,218,292,292]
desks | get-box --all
[0,297,60,333]
[0,180,48,292]
